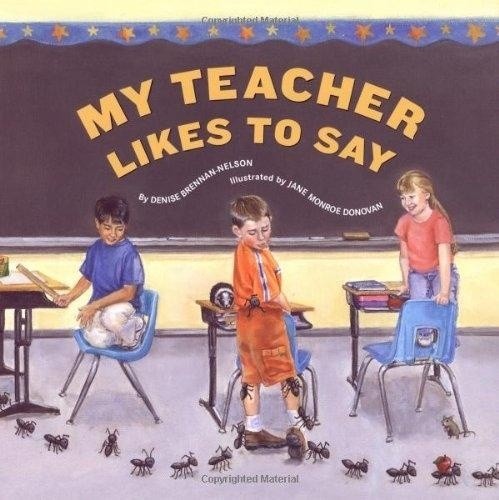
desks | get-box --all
[0,269,70,420]
[193,298,317,422]
[344,279,452,399]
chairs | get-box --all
[219,312,322,433]
[59,291,162,425]
[347,298,472,444]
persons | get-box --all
[226,194,311,463]
[52,195,149,350]
[394,169,462,349]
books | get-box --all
[215,311,310,332]
[346,280,390,312]
